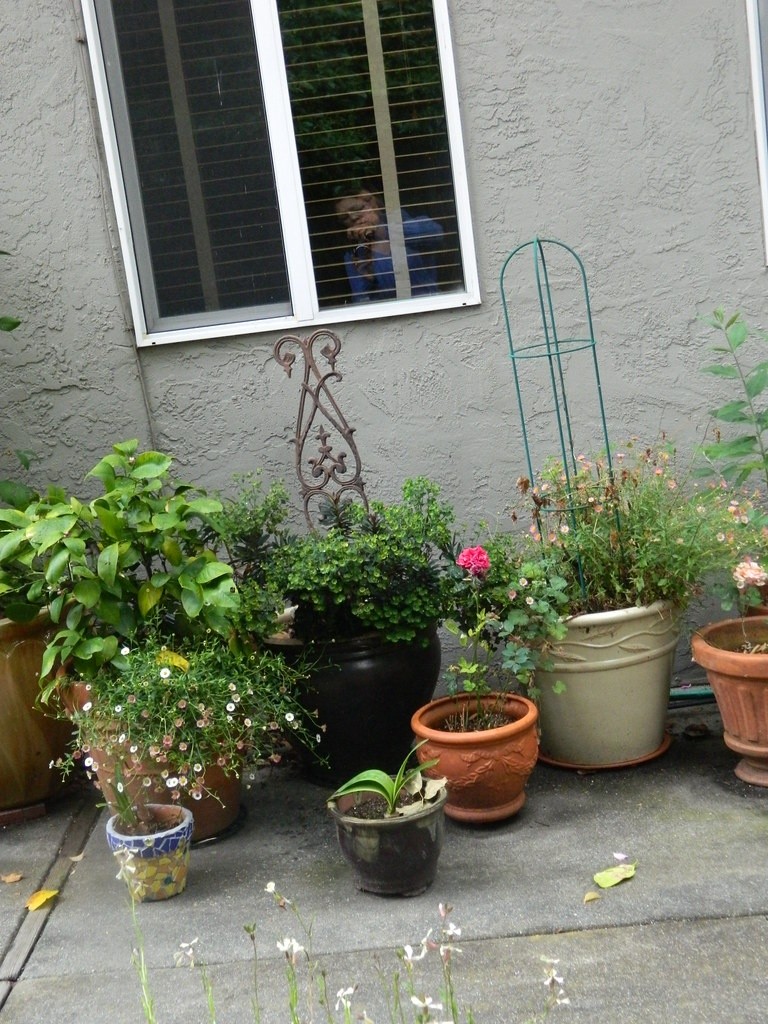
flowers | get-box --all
[435,549,517,724]
[699,307,768,646]
[1,445,339,804]
[457,436,740,700]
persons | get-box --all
[333,184,441,303]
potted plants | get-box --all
[198,461,516,789]
[105,759,193,902]
[328,738,448,899]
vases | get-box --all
[57,653,244,850]
[506,599,682,770]
[691,616,768,788]
[735,583,768,620]
[410,692,540,825]
